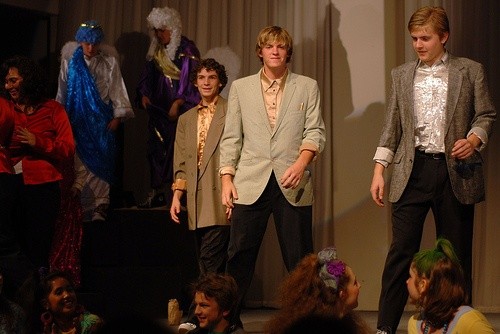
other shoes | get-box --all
[138,189,158,208]
[226,319,243,334]
[376,328,390,334]
[91,205,108,221]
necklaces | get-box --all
[424,320,448,334]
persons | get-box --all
[0,57,76,257]
[406,238,497,334]
[261,248,368,334]
[0,274,28,334]
[179,272,248,334]
[369,7,497,334]
[170,58,232,276]
[34,264,108,334]
[218,26,326,307]
[55,21,134,222]
[134,6,201,209]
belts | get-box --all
[415,152,446,160]
